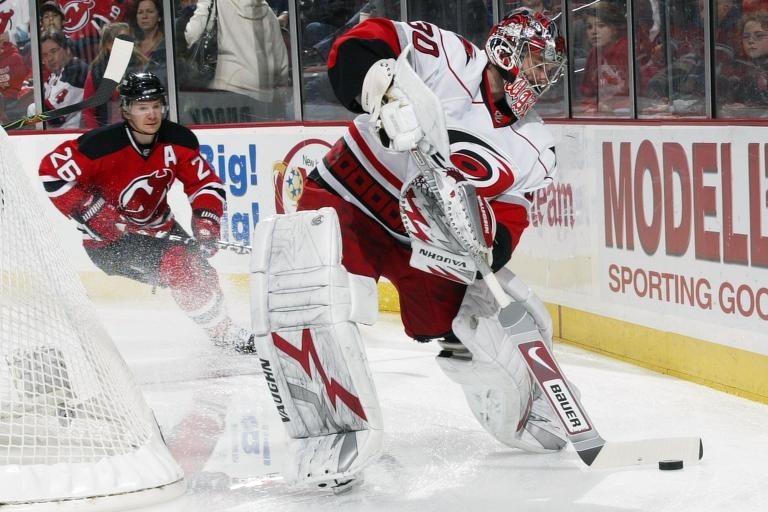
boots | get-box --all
[298,433,370,485]
[511,412,566,455]
[208,318,253,352]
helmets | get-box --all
[115,71,167,101]
[485,7,566,85]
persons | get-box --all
[0,0,164,129]
[581,0,767,109]
[466,1,491,49]
[248,10,572,497]
[40,72,256,358]
[171,1,395,105]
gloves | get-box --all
[190,204,222,259]
[79,191,124,245]
[375,66,436,156]
[409,168,490,267]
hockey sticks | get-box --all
[0,35,134,131]
[383,95,704,470]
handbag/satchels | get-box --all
[183,37,217,87]
